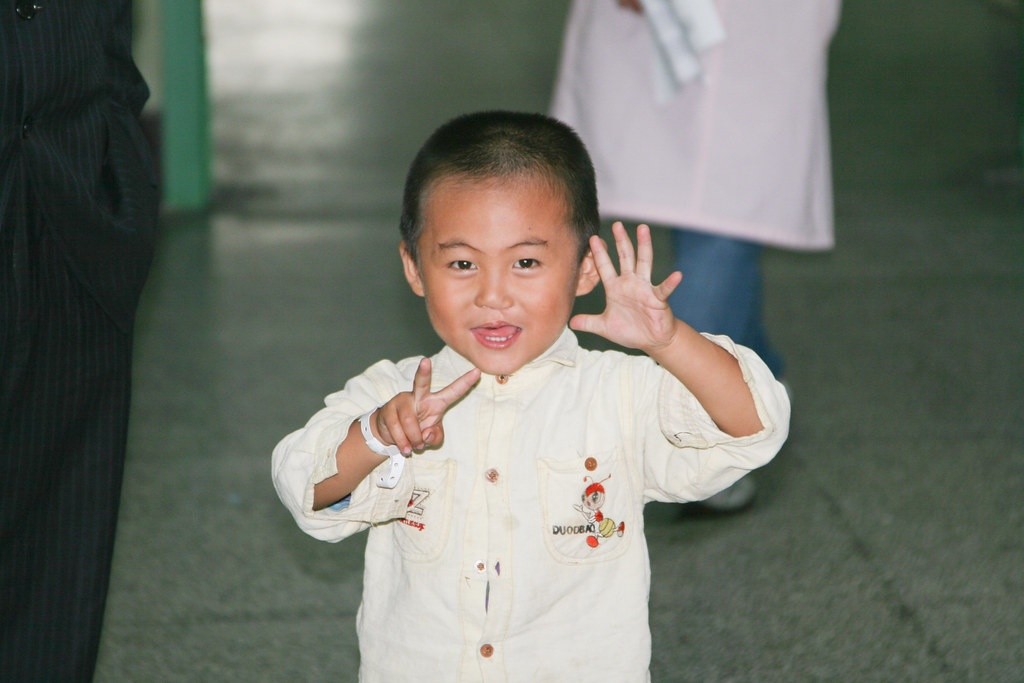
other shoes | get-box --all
[698,474,759,511]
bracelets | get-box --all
[357,408,408,488]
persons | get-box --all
[271,110,790,683]
[547,0,837,524]
[0,0,168,683]
